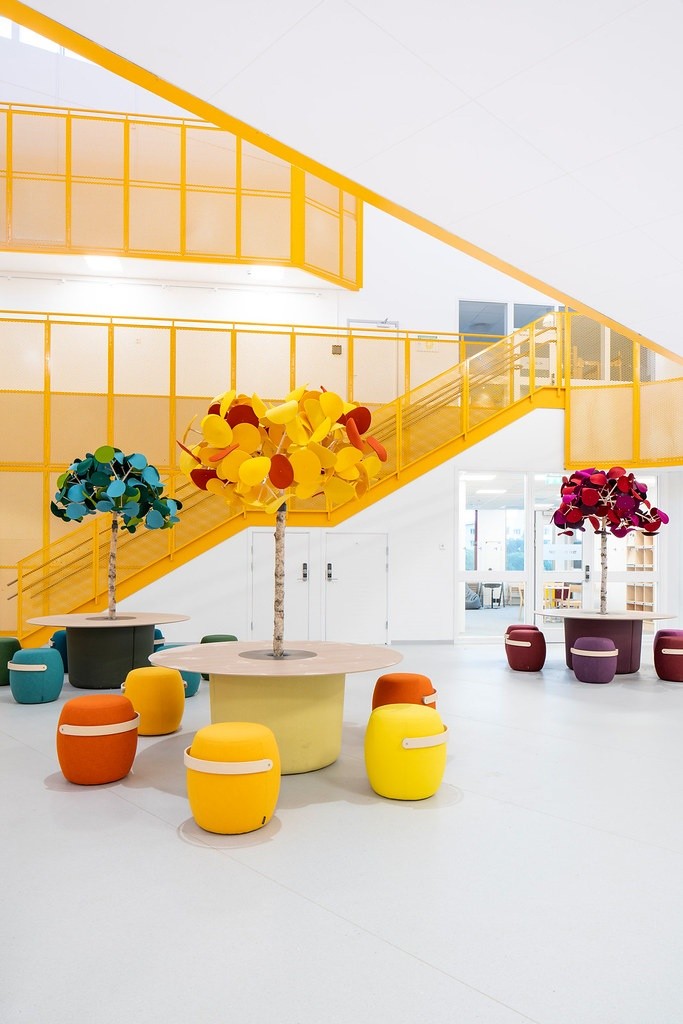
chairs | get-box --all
[559,584,583,622]
[544,581,565,622]
[509,582,524,605]
[518,585,552,623]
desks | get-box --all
[546,587,570,622]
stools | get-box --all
[364,704,448,800]
[48,630,68,673]
[57,695,141,785]
[653,629,683,652]
[183,722,282,835]
[505,625,538,654]
[121,667,187,737]
[506,630,547,671]
[570,637,618,683]
[154,629,165,653]
[0,638,22,687]
[372,673,438,712]
[7,647,64,704]
[201,634,238,682]
[156,645,201,697]
[654,636,683,682]
